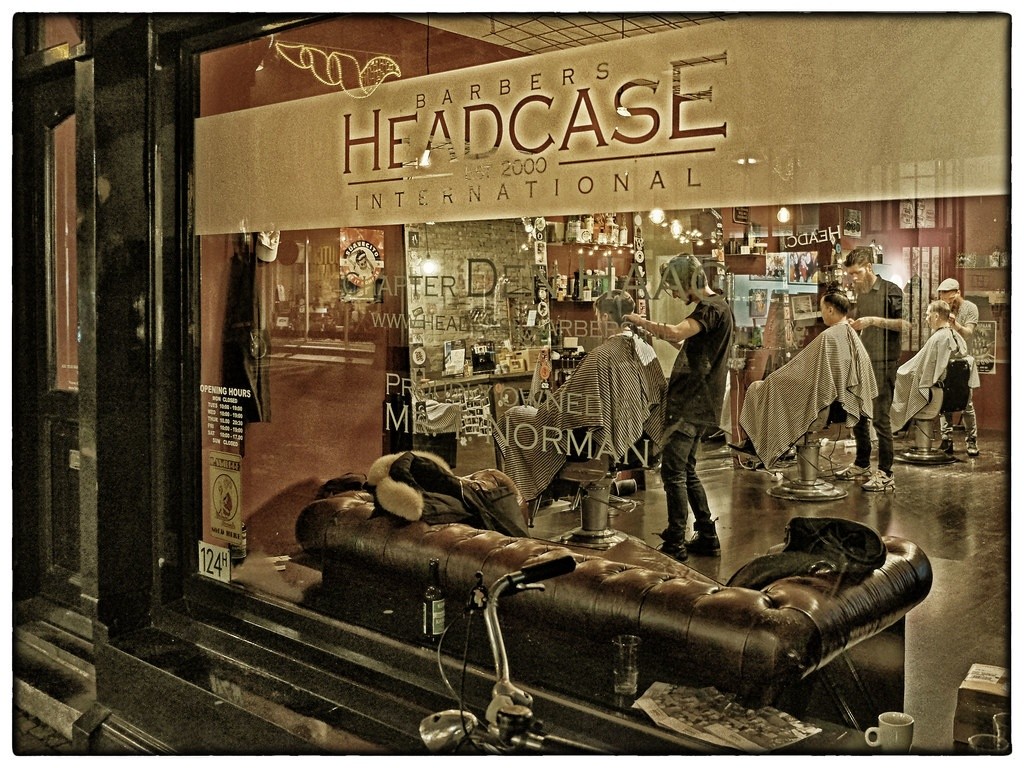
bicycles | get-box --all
[419,556,618,756]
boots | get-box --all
[684,517,721,557]
[652,528,688,561]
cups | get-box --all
[611,635,640,694]
[865,712,914,753]
[968,711,1009,751]
[585,218,594,234]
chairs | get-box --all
[766,397,850,500]
[547,426,645,549]
[891,359,971,466]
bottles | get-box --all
[620,228,627,244]
[422,558,446,645]
[561,275,567,287]
[582,288,591,301]
[729,233,736,254]
[753,243,767,254]
[557,288,563,301]
[605,218,619,244]
[227,521,246,565]
[992,248,1008,267]
[587,270,593,296]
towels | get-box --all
[608,326,658,371]
[425,401,452,421]
[962,354,982,388]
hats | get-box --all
[936,278,959,291]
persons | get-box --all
[623,252,736,560]
[789,251,815,282]
[936,278,979,457]
[356,251,373,278]
[837,248,912,491]
[728,293,851,453]
[494,290,635,500]
[889,300,951,435]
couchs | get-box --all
[294,470,934,729]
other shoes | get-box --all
[938,440,954,454]
[727,439,759,456]
[965,437,980,456]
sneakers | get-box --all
[835,463,872,481]
[861,469,896,491]
